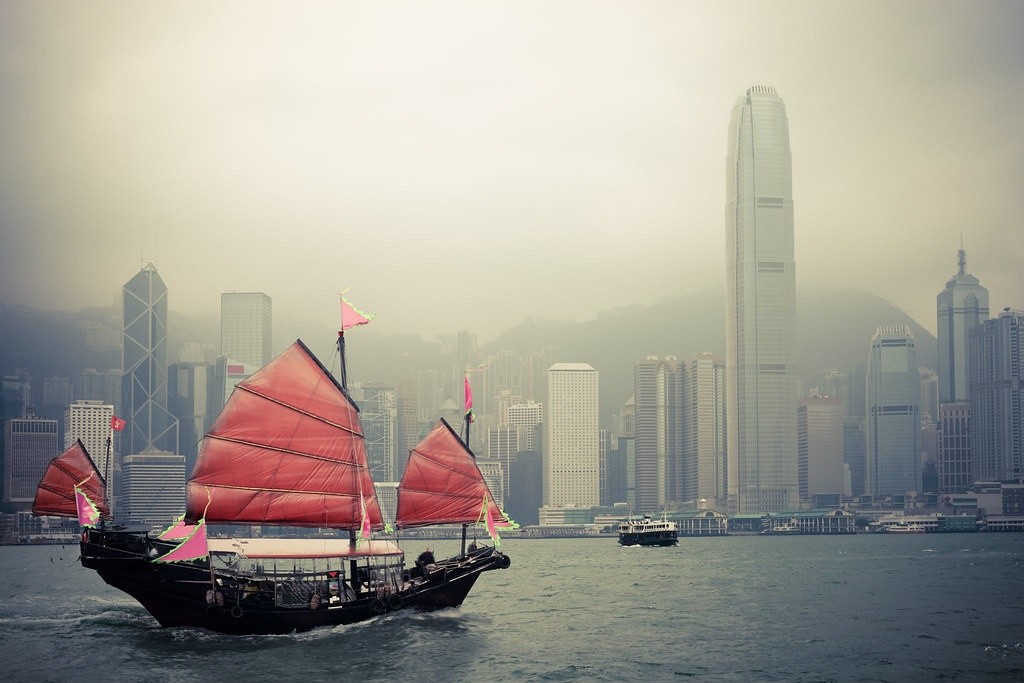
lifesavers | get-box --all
[386,593,403,611]
[229,603,246,618]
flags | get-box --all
[111,416,128,431]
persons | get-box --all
[418,547,435,578]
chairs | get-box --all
[415,561,428,580]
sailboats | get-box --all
[76,296,520,636]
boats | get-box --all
[617,510,680,547]
